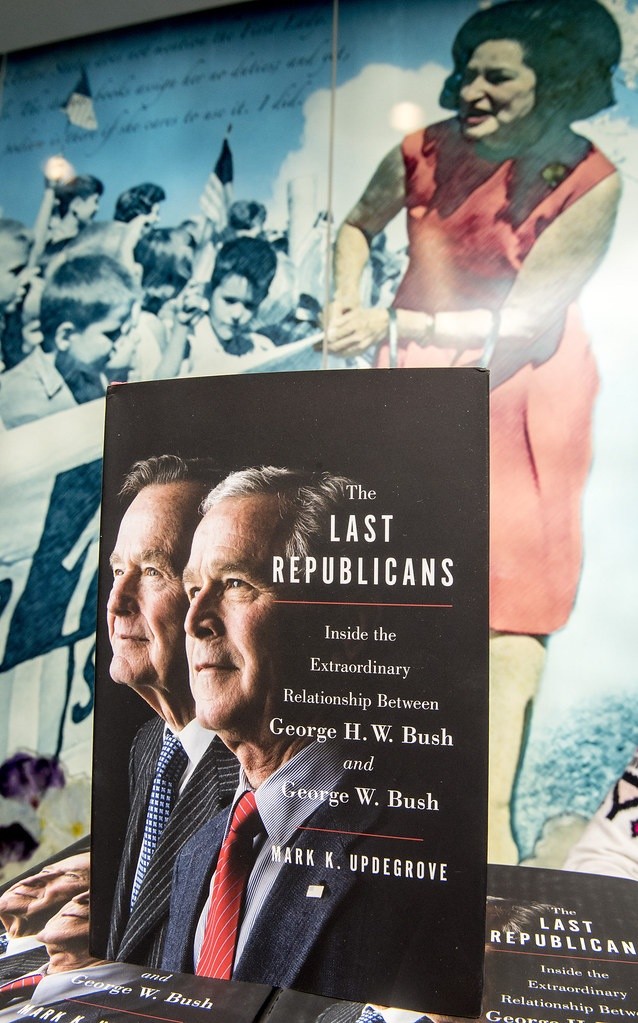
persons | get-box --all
[107,454,490,1019]
[320,0,622,867]
[0,174,408,430]
[0,846,638,1023]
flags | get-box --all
[199,138,235,234]
[59,70,98,131]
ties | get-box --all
[196,789,267,977]
[130,727,188,915]
[0,974,47,1004]
[0,936,9,954]
